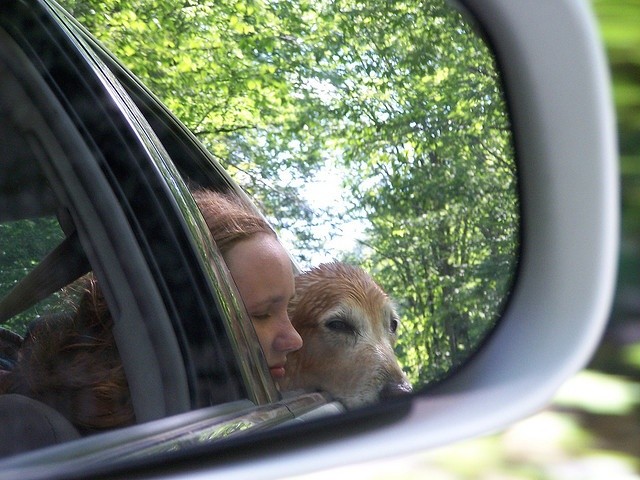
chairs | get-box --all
[0,392,83,457]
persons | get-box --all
[1,182,305,428]
[15,310,136,427]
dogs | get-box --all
[273,257,415,413]
